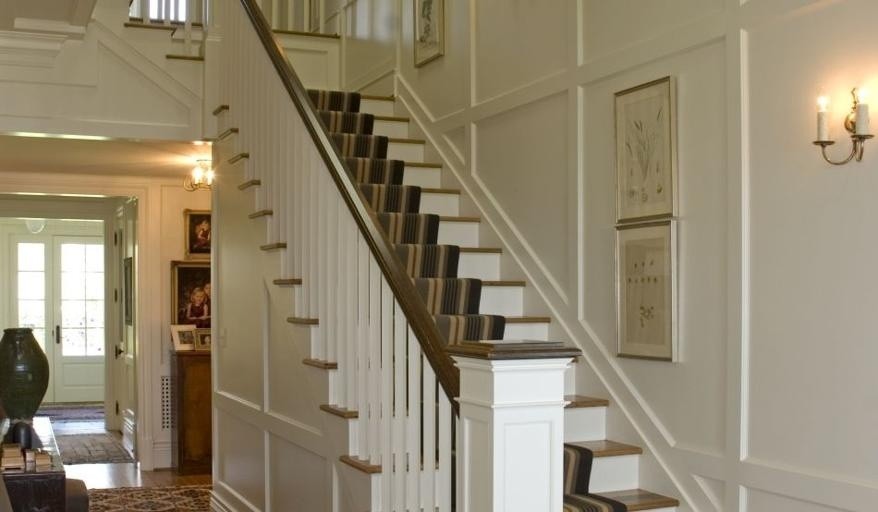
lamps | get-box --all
[183,159,212,192]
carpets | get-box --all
[88,484,214,512]
[38,405,104,420]
[300,90,627,512]
[55,433,134,464]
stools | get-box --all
[65,480,88,512]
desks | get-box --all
[0,416,65,512]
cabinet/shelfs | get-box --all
[170,351,211,475]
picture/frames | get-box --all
[614,74,678,362]
[183,209,210,260]
[413,0,444,67]
[171,260,210,351]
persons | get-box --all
[194,219,211,252]
[179,279,211,324]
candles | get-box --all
[856,104,869,135]
[817,110,828,141]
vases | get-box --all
[0,328,49,424]
